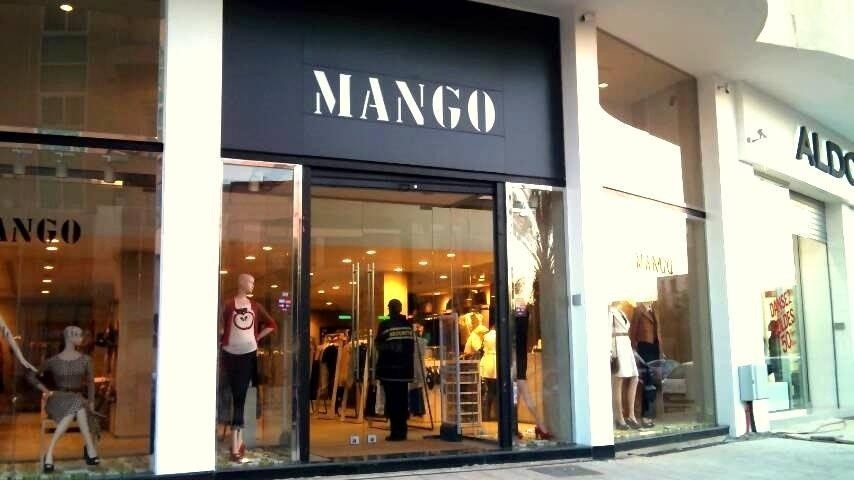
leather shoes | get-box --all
[385,430,408,441]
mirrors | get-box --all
[314,333,344,419]
[341,328,372,424]
[406,340,434,430]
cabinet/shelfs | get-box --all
[446,359,482,436]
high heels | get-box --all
[229,442,247,463]
[84,444,101,465]
[43,454,55,474]
[615,418,656,431]
[518,432,523,440]
[535,424,553,441]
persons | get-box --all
[609,296,642,430]
[464,325,489,354]
[512,300,553,439]
[220,273,278,460]
[768,321,781,378]
[478,324,498,420]
[628,293,666,426]
[374,298,414,441]
[25,325,101,472]
[415,324,431,345]
[0,313,36,371]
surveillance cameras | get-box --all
[724,83,730,94]
[757,129,769,137]
[582,14,593,23]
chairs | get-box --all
[40,389,89,469]
[661,362,695,418]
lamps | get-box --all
[248,171,259,192]
[6,148,130,183]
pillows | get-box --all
[667,360,694,379]
